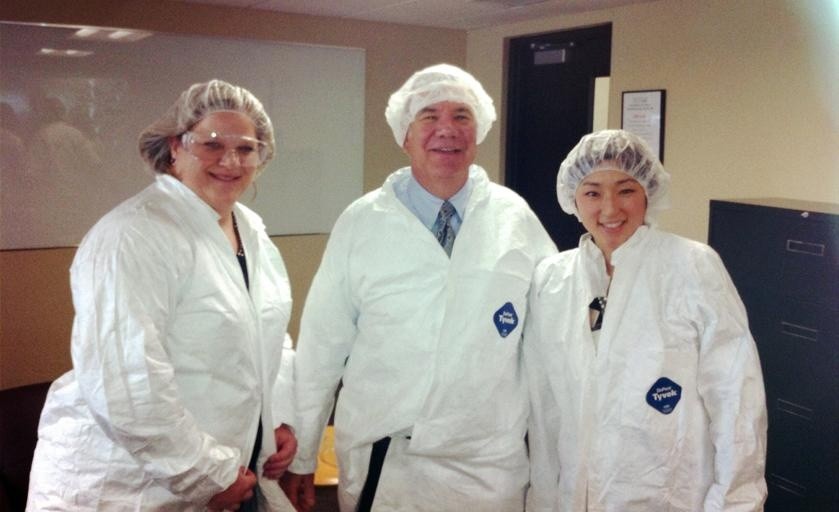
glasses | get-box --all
[181,130,269,167]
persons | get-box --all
[278,64,562,510]
[26,80,297,511]
[524,129,769,512]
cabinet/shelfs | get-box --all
[706,193,839,511]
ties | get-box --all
[431,202,456,257]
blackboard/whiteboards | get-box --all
[0,19,364,248]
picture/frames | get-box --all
[621,88,666,167]
